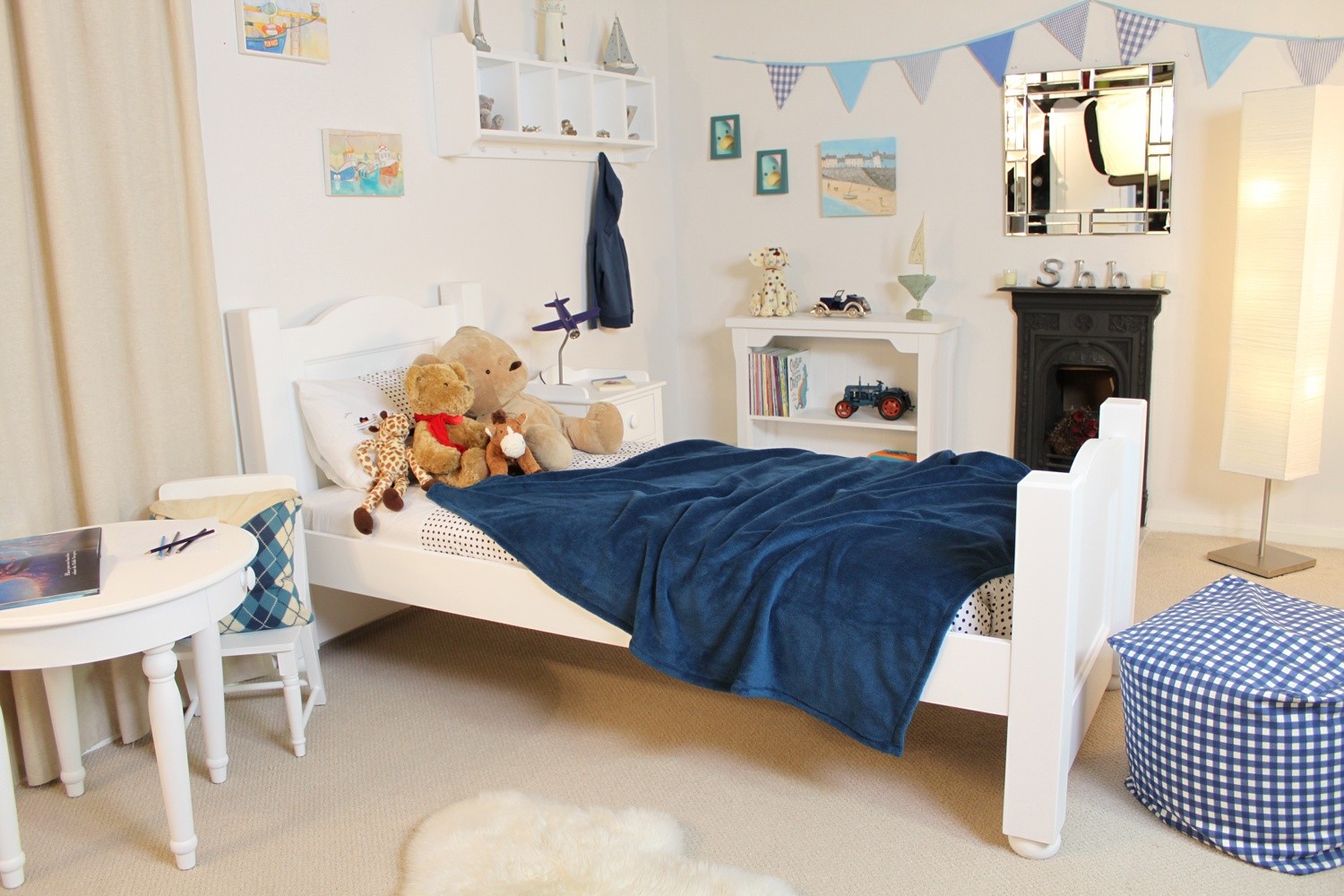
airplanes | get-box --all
[532,291,602,340]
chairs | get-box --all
[159,473,329,758]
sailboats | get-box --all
[459,0,492,52]
[602,12,639,77]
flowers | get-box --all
[1066,411,1105,445]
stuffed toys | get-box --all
[746,245,798,318]
[348,324,624,539]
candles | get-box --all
[1003,269,1017,285]
[1151,272,1166,290]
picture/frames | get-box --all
[757,149,789,195]
[710,114,742,159]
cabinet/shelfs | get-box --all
[728,317,960,458]
[435,33,656,161]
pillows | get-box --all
[148,488,314,637]
[291,367,408,490]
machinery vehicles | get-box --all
[834,375,916,421]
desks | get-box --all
[0,520,260,888]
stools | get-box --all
[1106,574,1344,877]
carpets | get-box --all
[395,793,791,895]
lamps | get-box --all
[533,291,602,385]
[1207,84,1344,578]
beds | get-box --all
[224,282,1150,862]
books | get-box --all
[0,527,102,609]
[748,345,813,417]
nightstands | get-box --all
[524,380,667,443]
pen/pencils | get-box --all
[157,535,164,559]
[176,528,207,554]
[165,531,179,556]
[144,529,214,554]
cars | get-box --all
[809,290,872,318]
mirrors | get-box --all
[1002,60,1178,239]
